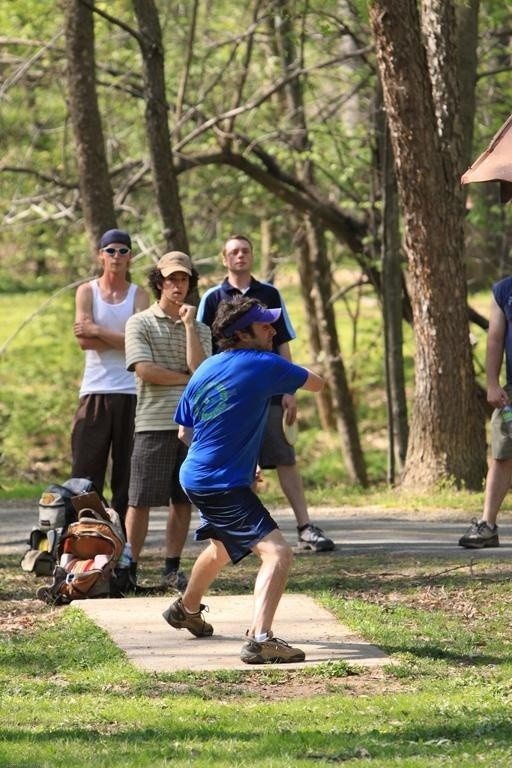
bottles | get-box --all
[499,396,512,440]
[119,543,132,571]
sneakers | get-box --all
[163,598,213,637]
[459,518,499,548]
[241,636,305,664]
[161,572,188,593]
[297,524,336,552]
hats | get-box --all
[157,252,192,277]
[225,303,282,331]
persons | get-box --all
[195,235,335,552]
[113,252,213,592]
[459,275,512,548]
[161,297,325,663]
[73,231,149,542]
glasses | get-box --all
[100,247,132,255]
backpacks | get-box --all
[37,507,133,604]
[21,478,101,576]
